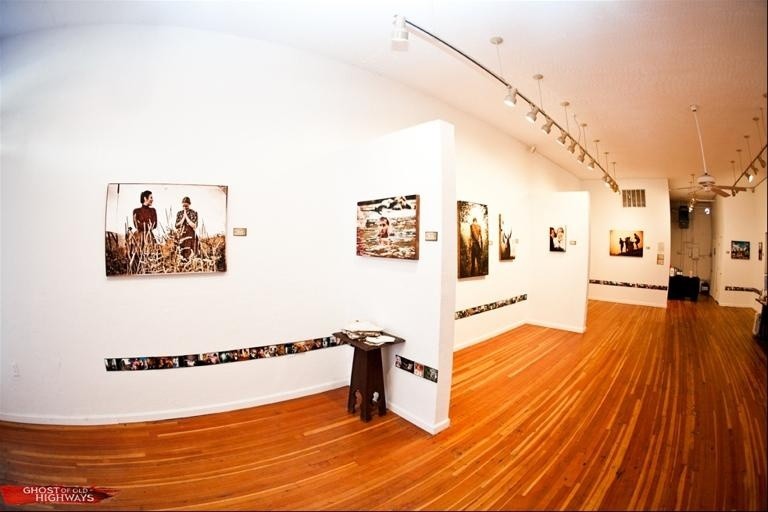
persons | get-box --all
[378,217,394,240]
[133,190,157,242]
[468,218,483,274]
[550,227,564,251]
[174,198,198,258]
[619,233,640,252]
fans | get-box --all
[665,105,747,198]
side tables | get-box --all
[332,330,405,422]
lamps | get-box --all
[403,20,620,193]
[730,93,767,197]
